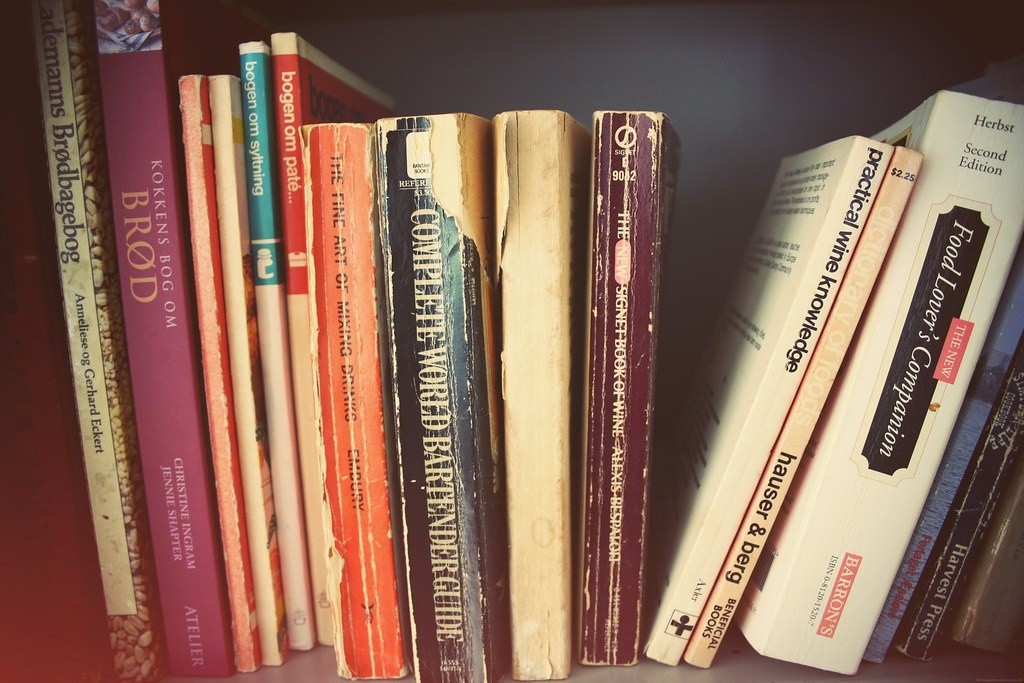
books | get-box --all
[33,1,1023,681]
